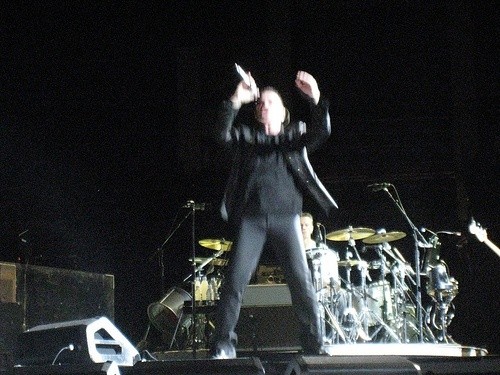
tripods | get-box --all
[326,231,431,345]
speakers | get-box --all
[0,260,422,375]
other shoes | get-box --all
[206,345,236,359]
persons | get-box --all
[208,71,338,359]
[300,213,328,250]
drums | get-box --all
[148,286,193,339]
[350,281,394,327]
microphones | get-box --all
[185,202,213,210]
[367,183,391,192]
[234,63,251,89]
[317,223,323,242]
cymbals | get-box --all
[326,227,376,241]
[189,256,226,267]
[362,231,406,244]
[198,239,233,251]
[338,259,359,267]
